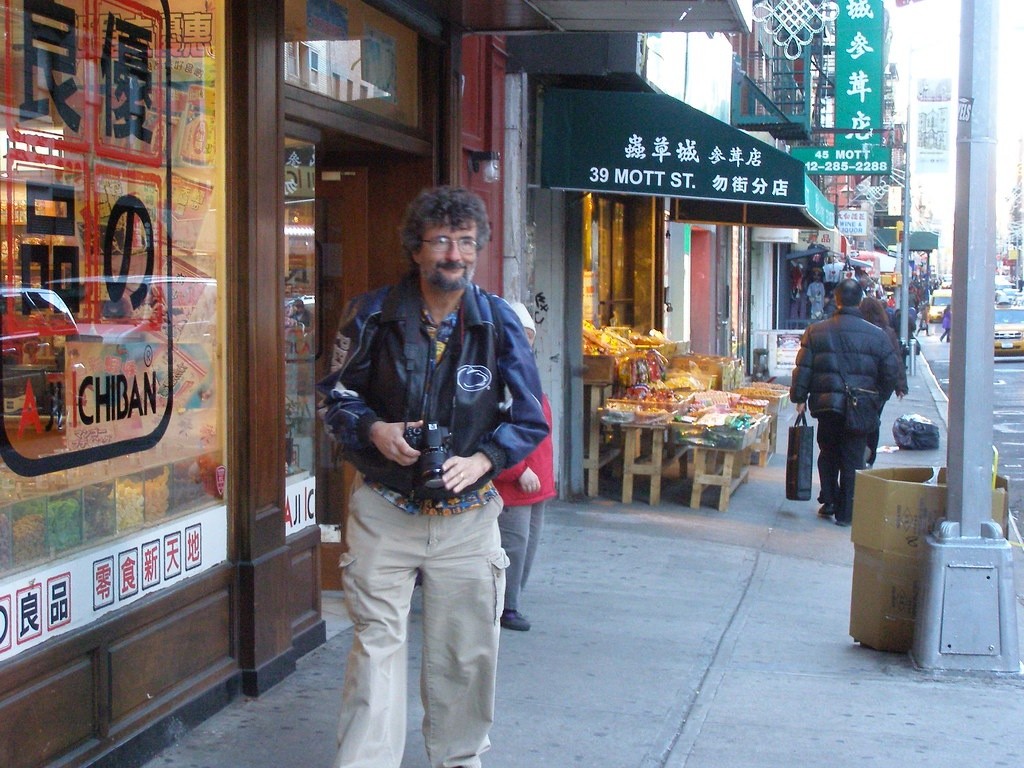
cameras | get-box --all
[403,421,453,488]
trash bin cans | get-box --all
[849,466,1009,654]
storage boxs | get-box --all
[851,467,1010,653]
[584,339,791,450]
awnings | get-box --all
[541,87,835,232]
[847,257,873,269]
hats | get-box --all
[509,302,537,337]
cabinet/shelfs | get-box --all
[584,384,777,511]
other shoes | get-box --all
[502,608,531,632]
[818,502,852,528]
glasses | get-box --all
[423,237,478,254]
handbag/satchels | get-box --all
[787,413,814,502]
[920,319,927,330]
[911,335,921,355]
[843,383,880,432]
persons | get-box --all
[939,305,951,343]
[498,302,556,631]
[316,189,550,768]
[790,279,917,526]
[916,304,930,337]
